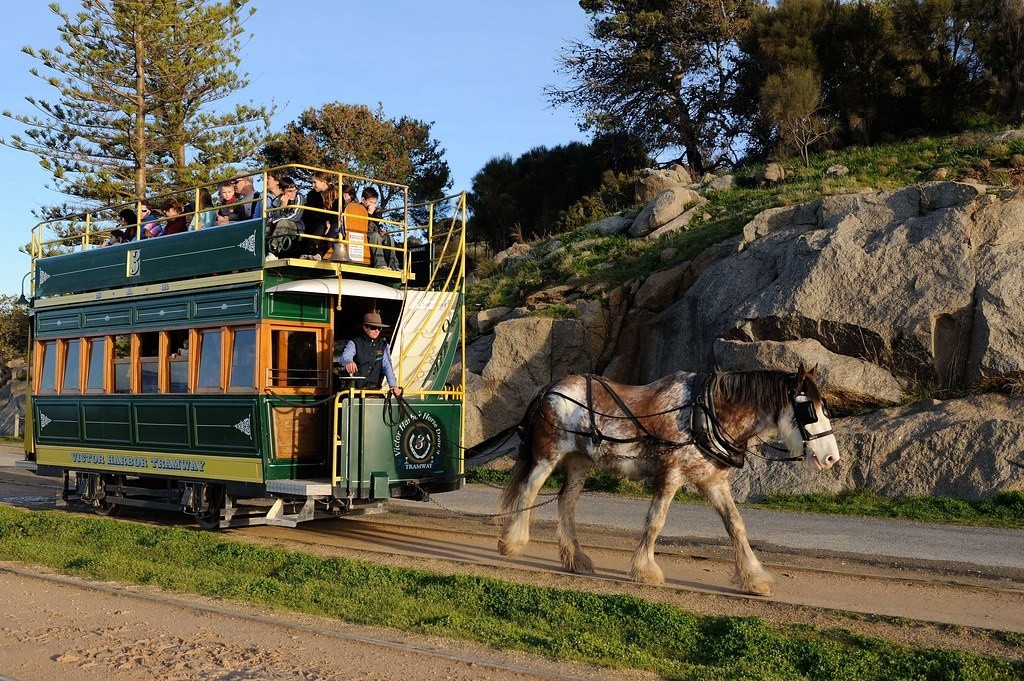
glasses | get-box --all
[142,209,150,213]
[349,193,355,202]
[367,325,382,331]
[235,180,246,184]
[285,189,298,194]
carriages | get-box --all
[23,162,841,597]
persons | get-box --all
[171,338,189,358]
[109,167,401,271]
[338,314,401,399]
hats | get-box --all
[231,171,253,182]
[363,313,390,328]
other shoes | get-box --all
[266,244,276,254]
[379,265,392,270]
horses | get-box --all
[496,362,840,597]
